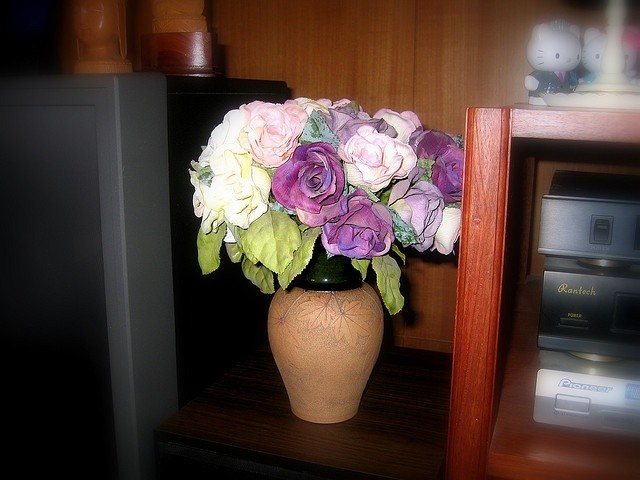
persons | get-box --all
[73,0,132,75]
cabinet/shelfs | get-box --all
[443,106,638,477]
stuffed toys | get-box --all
[585,26,640,86]
[525,21,583,105]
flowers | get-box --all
[187,97,464,315]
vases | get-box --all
[266,235,385,425]
